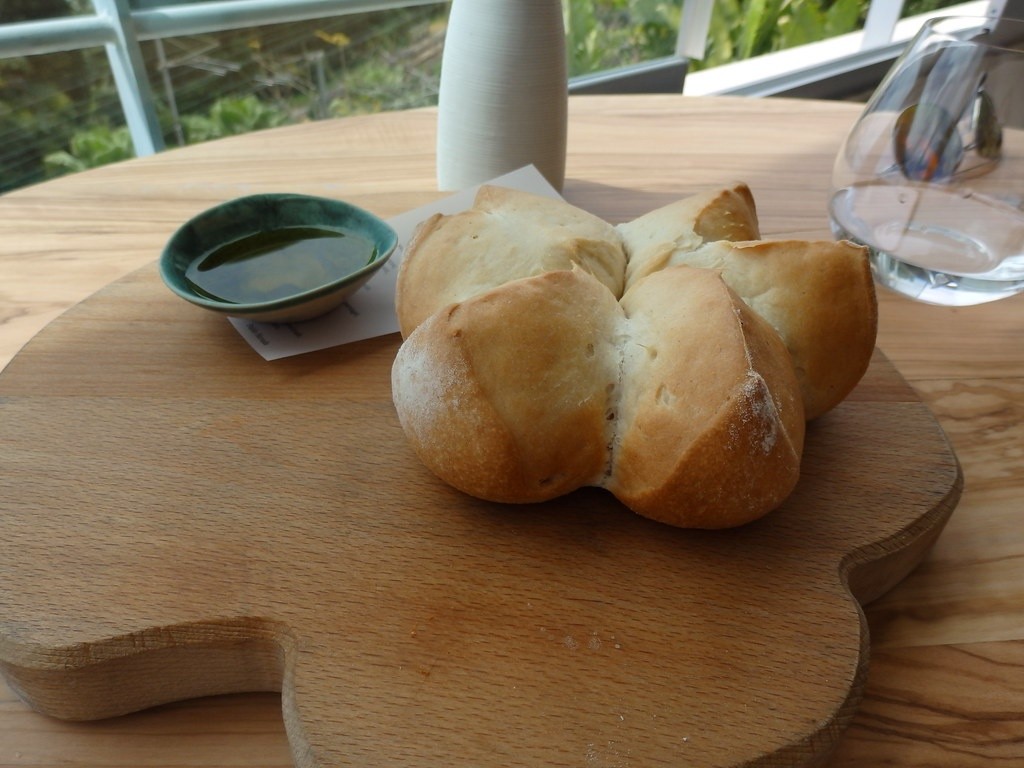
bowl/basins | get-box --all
[156,189,398,326]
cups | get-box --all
[827,16,1024,307]
[434,1,569,205]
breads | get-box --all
[388,179,878,529]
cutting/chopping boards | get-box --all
[1,190,962,768]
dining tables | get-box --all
[0,96,1024,768]
